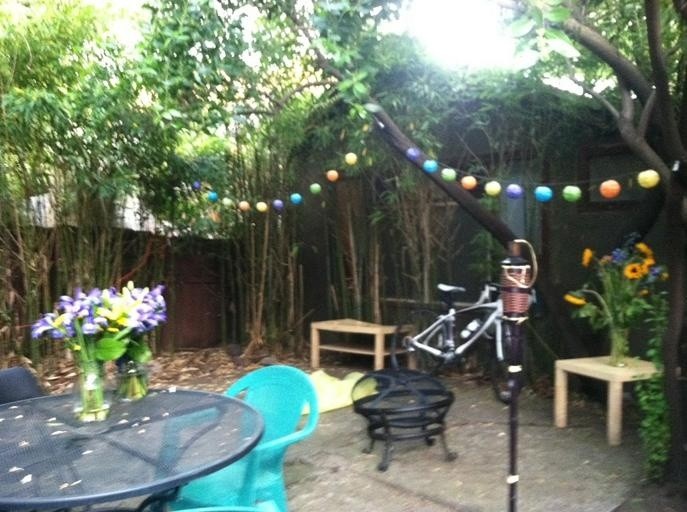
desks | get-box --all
[552,354,665,446]
[309,317,417,373]
[1,387,264,512]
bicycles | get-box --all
[389,283,535,404]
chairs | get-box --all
[155,363,320,510]
[0,369,92,512]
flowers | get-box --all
[29,278,168,412]
[559,238,670,356]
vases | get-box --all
[604,327,633,367]
[77,355,150,422]
